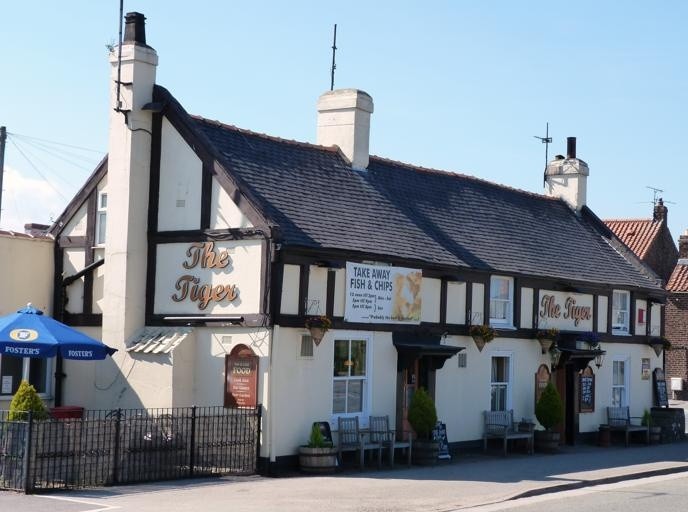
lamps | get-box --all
[590,335,608,368]
[549,344,562,371]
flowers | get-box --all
[470,324,497,341]
[538,325,557,340]
[649,334,672,351]
[303,315,331,330]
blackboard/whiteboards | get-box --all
[430,424,448,455]
[656,381,668,406]
[579,377,595,410]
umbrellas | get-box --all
[0,301,119,381]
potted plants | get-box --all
[639,408,668,443]
[297,419,338,472]
[537,376,568,456]
[406,385,440,464]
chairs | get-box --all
[478,407,536,458]
[336,414,385,470]
[603,403,651,446]
[369,413,413,465]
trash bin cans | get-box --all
[599,424,611,449]
[650,406,686,443]
